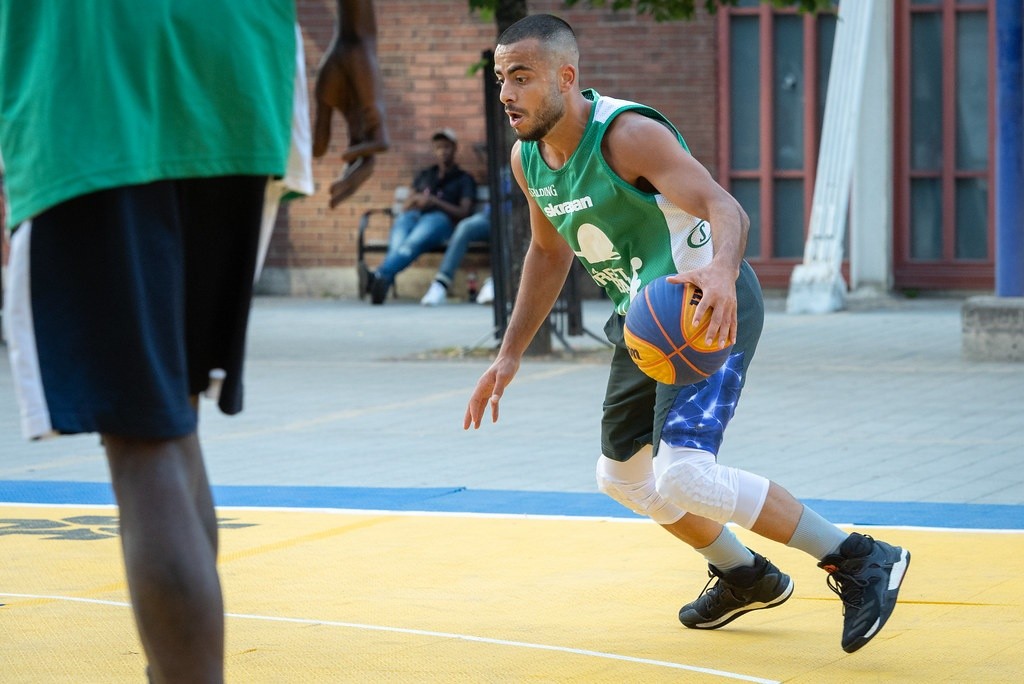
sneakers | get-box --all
[678,546,795,629]
[816,531,910,654]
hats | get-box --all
[430,126,457,144]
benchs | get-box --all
[358,208,491,300]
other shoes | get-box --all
[372,279,389,305]
[477,279,495,304]
[357,263,374,295]
[421,282,446,305]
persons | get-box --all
[464,13,912,654]
[356,128,478,305]
[0,0,388,684]
[420,160,518,305]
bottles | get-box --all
[464,267,479,303]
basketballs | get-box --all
[624,273,734,386]
